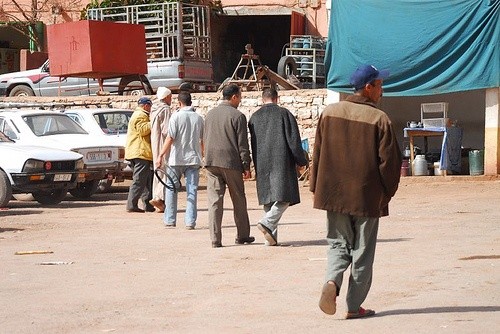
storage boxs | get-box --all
[423,118,449,127]
[423,102,450,113]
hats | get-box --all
[157,87,171,100]
[350,64,389,89]
[137,97,152,104]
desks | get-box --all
[403,126,463,175]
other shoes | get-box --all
[235,236,255,245]
[144,200,155,212]
[186,226,196,230]
[165,225,176,228]
[257,223,277,245]
[129,207,145,213]
[212,241,222,247]
[149,198,165,212]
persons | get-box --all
[155,90,204,230]
[203,84,255,248]
[149,87,171,213]
[124,97,156,213]
[309,65,402,319]
[248,88,309,245]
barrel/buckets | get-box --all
[400,145,427,176]
[469,149,484,176]
[434,161,444,175]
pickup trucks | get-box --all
[0,56,214,97]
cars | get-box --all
[0,101,135,208]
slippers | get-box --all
[345,307,375,318]
[318,283,336,315]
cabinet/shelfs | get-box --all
[285,34,327,89]
